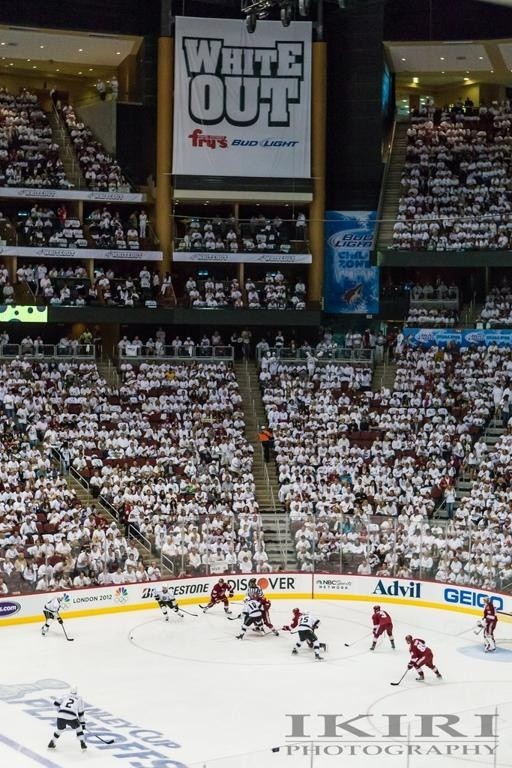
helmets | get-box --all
[406,634,412,641]
[162,586,168,591]
[58,595,63,600]
[70,685,76,694]
[219,578,224,582]
[373,605,380,610]
[250,578,256,582]
[293,608,299,614]
[243,592,258,602]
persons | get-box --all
[248,578,264,599]
[40,596,63,635]
[236,598,265,640]
[1,75,512,309]
[2,311,512,596]
[251,591,279,636]
[477,597,498,652]
[203,578,234,615]
[155,585,184,622]
[405,635,441,680]
[48,686,88,749]
[284,608,326,661]
[370,605,395,651]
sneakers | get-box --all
[42,627,46,635]
[236,626,279,638]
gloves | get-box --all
[57,616,63,624]
[408,662,414,669]
[154,595,159,600]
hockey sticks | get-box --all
[227,617,239,620]
[57,612,74,641]
[474,627,483,635]
[199,597,230,609]
[178,608,198,616]
[390,668,409,685]
[85,728,114,744]
[344,632,371,647]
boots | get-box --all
[203,607,232,614]
[416,671,442,680]
[166,613,184,621]
[370,640,395,650]
[292,643,325,659]
[48,740,87,748]
